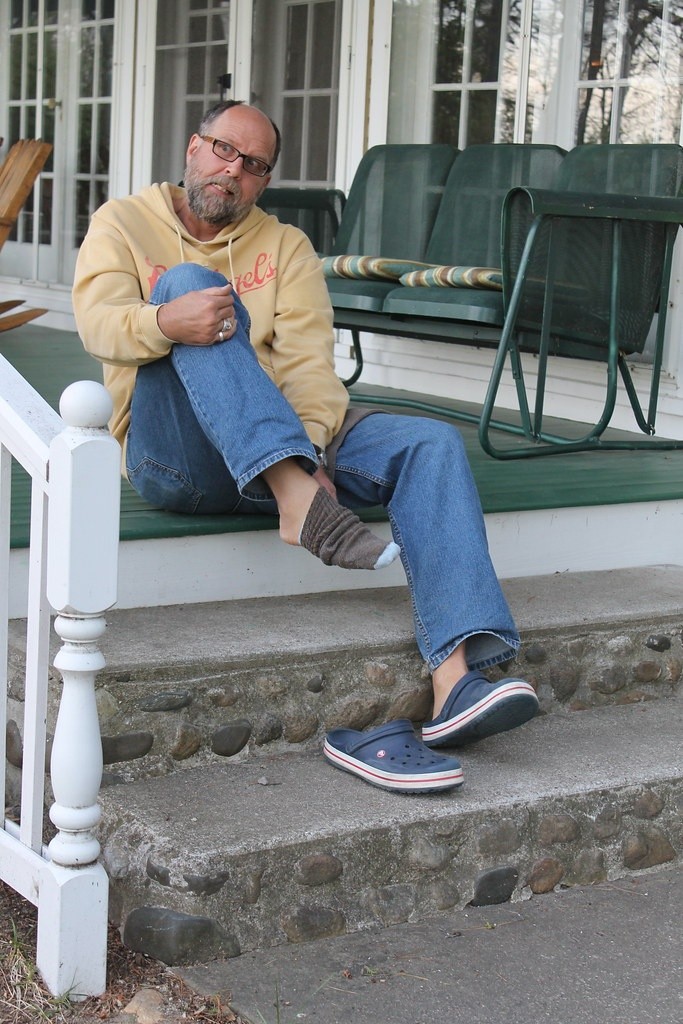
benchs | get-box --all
[257,142,683,458]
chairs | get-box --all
[0,137,54,335]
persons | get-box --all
[72,99,539,796]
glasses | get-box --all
[199,133,273,178]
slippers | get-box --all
[322,716,465,795]
[418,669,542,747]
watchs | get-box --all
[312,444,329,471]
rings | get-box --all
[221,318,232,332]
[218,332,225,341]
